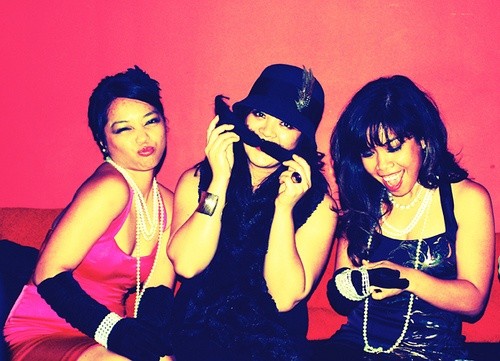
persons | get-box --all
[324,75,495,361]
[166,64,339,361]
[3,65,175,360]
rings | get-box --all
[291,172,302,184]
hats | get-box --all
[232,64,325,150]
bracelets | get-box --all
[94,312,122,349]
[198,191,220,218]
[334,267,370,302]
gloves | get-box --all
[37,270,176,361]
[326,267,409,317]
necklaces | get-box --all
[363,184,433,354]
[105,156,164,320]
